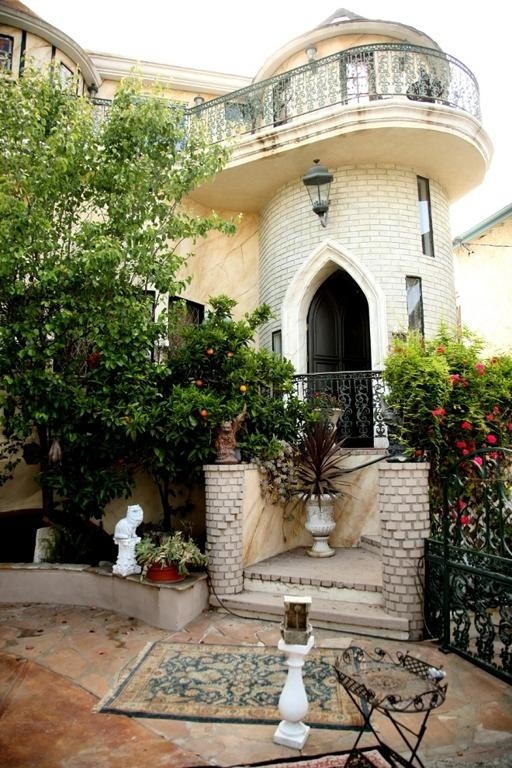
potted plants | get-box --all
[292,423,357,561]
[309,390,343,441]
[136,523,206,586]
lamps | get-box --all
[301,165,339,225]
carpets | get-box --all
[93,641,377,731]
